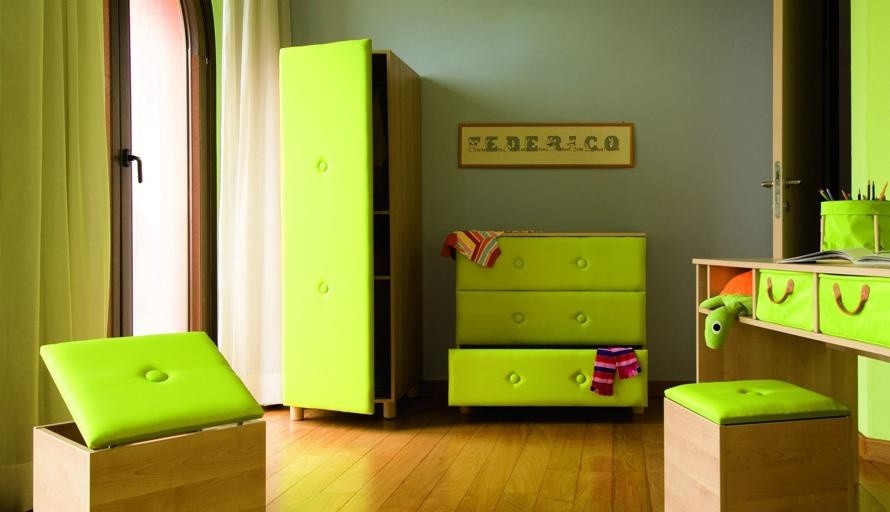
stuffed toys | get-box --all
[697,268,755,351]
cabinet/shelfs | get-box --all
[447,233,649,415]
[278,37,423,421]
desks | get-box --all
[691,258,890,384]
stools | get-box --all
[32,331,265,512]
[663,378,855,510]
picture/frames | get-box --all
[459,121,634,169]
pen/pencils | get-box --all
[817,180,889,201]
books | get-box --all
[775,247,890,267]
